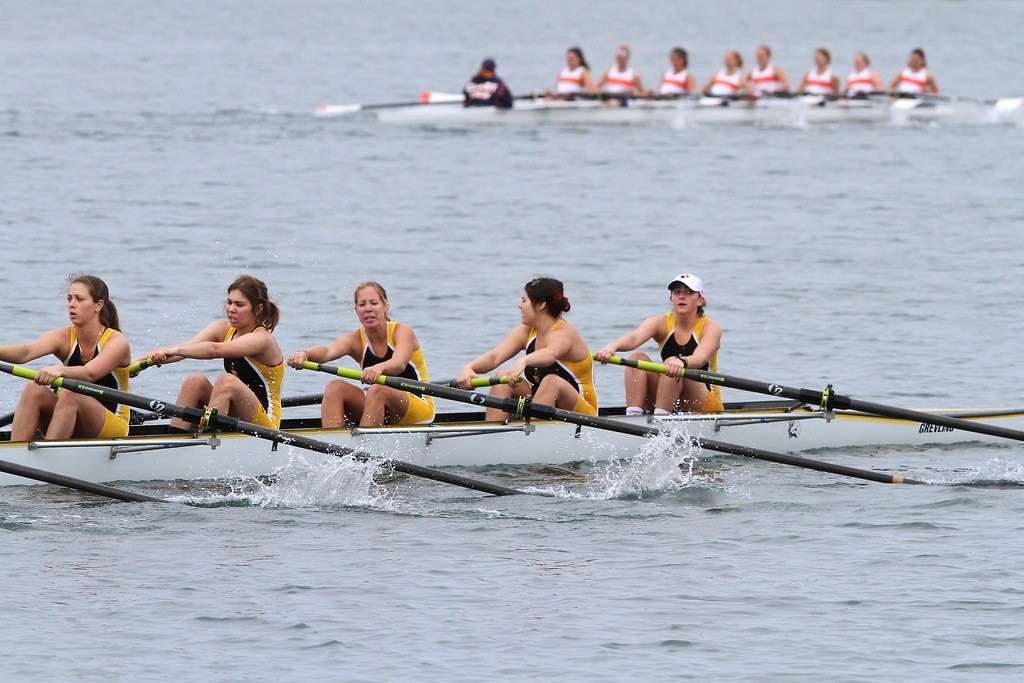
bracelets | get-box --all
[298,350,308,359]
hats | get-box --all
[667,274,704,297]
[482,59,494,70]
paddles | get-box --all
[595,354,1024,441]
[1,460,169,503]
[281,375,523,407]
[302,359,929,486]
[0,361,525,496]
[0,354,167,427]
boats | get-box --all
[377,98,1024,128]
[0,403,1024,488]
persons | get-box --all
[593,274,724,416]
[795,48,840,95]
[596,45,649,106]
[735,46,790,98]
[287,282,435,429]
[457,278,598,425]
[843,52,884,97]
[888,48,938,94]
[463,59,513,109]
[701,51,754,98]
[128,276,284,435]
[545,47,594,102]
[0,276,132,442]
[657,47,696,99]
